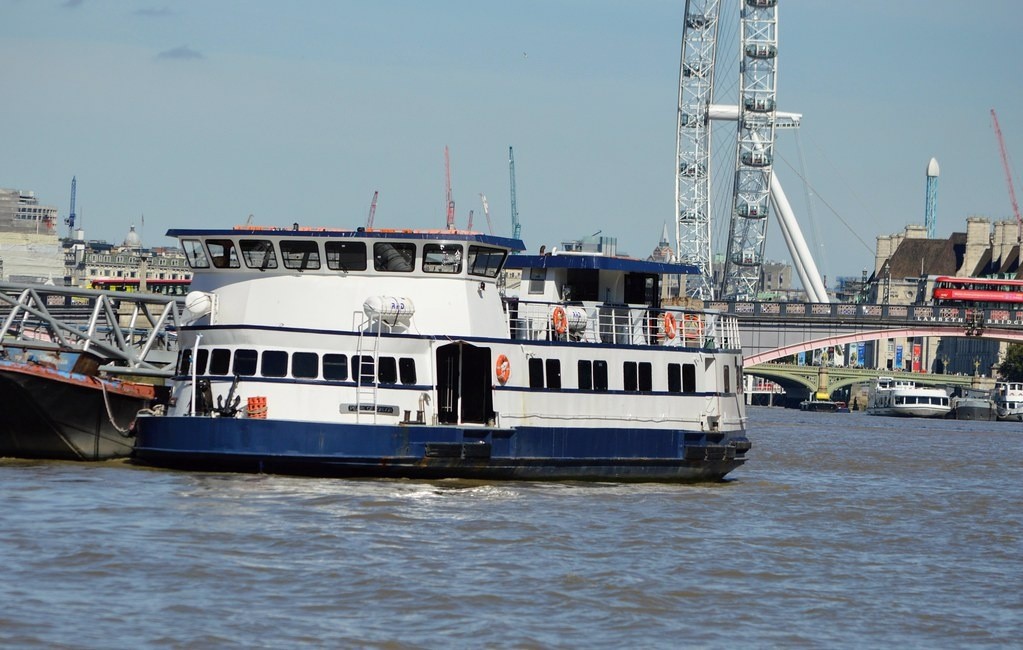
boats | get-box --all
[954,359,998,421]
[0,358,172,462]
[129,224,754,483]
[866,376,951,417]
[799,391,847,412]
[988,381,1023,422]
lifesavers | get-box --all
[680,316,704,340]
[496,355,510,383]
[665,313,676,339]
[553,306,566,334]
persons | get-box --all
[947,371,985,377]
[769,360,902,371]
